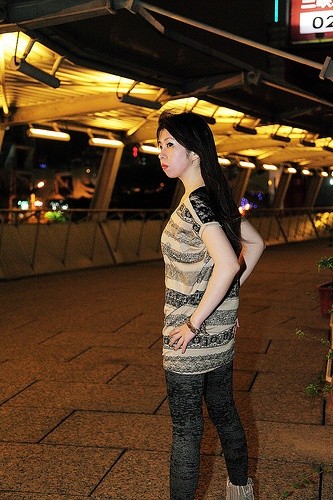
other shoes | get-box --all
[223,477,254,500]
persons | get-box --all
[156,111,266,500]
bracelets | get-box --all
[185,318,201,335]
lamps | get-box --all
[232,115,262,135]
[183,98,220,124]
[271,125,293,142]
[137,142,160,155]
[299,131,318,147]
[321,138,333,153]
[217,153,333,178]
[26,122,71,142]
[86,129,125,148]
[116,76,167,110]
[14,31,68,89]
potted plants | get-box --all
[316,256,333,319]
